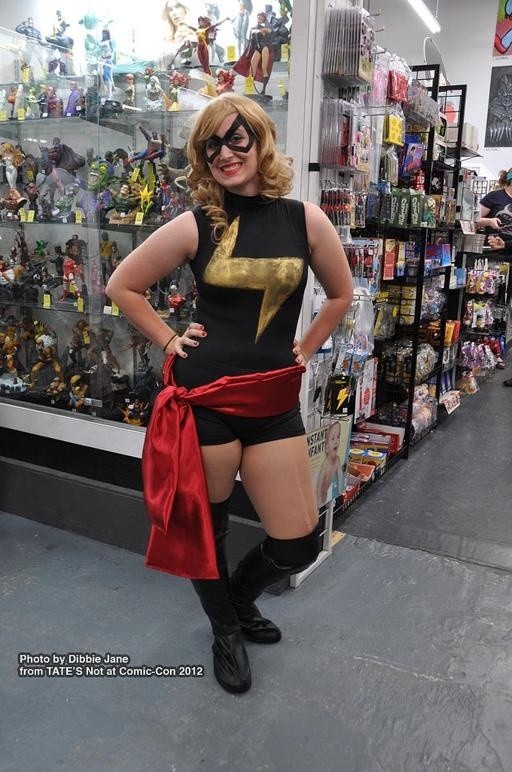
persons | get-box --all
[476,168,512,241]
[488,236,512,388]
[180,0,293,103]
[163,0,196,40]
[0,224,203,324]
[104,92,354,694]
[0,0,138,121]
[137,66,188,111]
[0,124,196,224]
[316,421,343,508]
[1,309,160,429]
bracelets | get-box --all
[162,333,178,352]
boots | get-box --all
[191,529,251,694]
[230,536,315,645]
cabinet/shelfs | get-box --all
[306,0,512,524]
[1,0,320,523]
